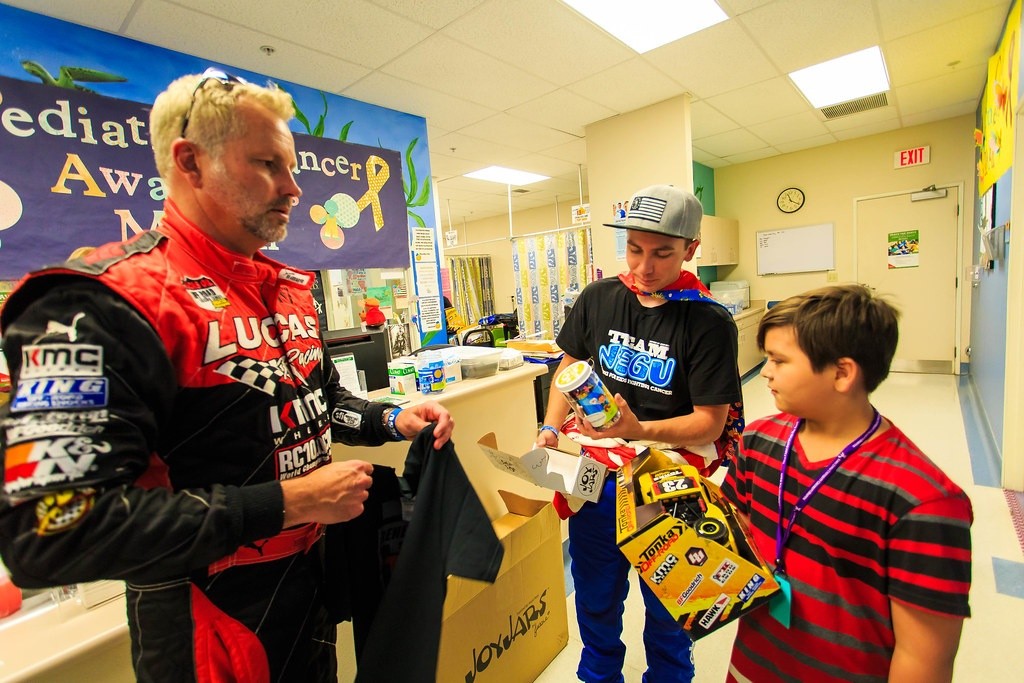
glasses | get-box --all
[179,66,248,139]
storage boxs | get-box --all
[434,489,570,683]
[477,433,608,503]
[388,345,524,395]
[616,447,782,641]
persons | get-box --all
[0,72,456,683]
[535,182,741,683]
[716,282,974,683]
[889,239,919,256]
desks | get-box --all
[332,365,548,522]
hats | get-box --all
[602,184,704,240]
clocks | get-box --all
[777,188,805,213]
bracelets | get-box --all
[540,425,560,440]
[386,408,410,441]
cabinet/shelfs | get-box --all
[696,215,740,267]
[734,308,765,382]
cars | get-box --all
[637,464,744,557]
